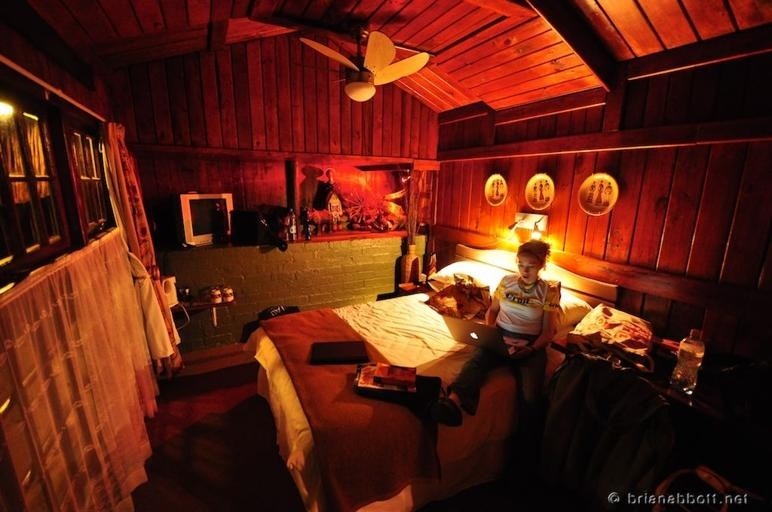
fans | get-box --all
[298,22,429,85]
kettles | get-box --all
[160,275,179,309]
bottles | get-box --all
[285,209,297,241]
[669,327,707,394]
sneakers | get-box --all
[438,398,462,427]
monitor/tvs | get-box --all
[178,193,234,248]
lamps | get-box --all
[503,212,548,240]
[344,68,377,102]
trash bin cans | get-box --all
[653,465,730,512]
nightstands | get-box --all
[397,280,428,293]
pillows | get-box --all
[558,289,591,326]
[429,260,514,296]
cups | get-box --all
[223,287,234,302]
[210,289,222,304]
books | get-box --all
[373,363,417,386]
[353,362,417,393]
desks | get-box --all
[552,331,747,426]
[174,293,234,354]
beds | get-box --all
[240,243,615,512]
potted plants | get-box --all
[400,170,424,283]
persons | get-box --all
[533,180,550,202]
[586,179,612,207]
[490,179,505,199]
[436,239,559,466]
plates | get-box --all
[577,173,619,216]
[525,173,555,210]
[484,174,508,207]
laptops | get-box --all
[443,314,529,357]
